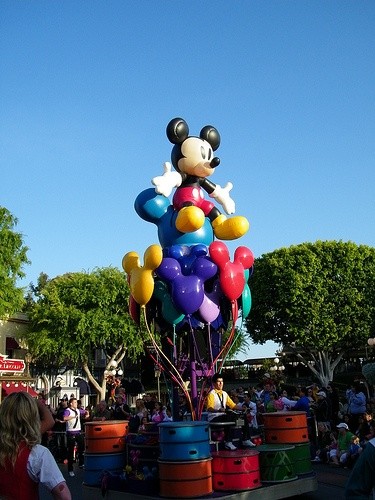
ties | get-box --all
[70,408,79,429]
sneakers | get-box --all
[224,442,237,451]
[242,439,256,448]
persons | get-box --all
[0,391,71,500]
[1,388,189,477]
[199,374,375,500]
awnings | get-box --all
[2,382,38,397]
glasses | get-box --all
[327,387,330,389]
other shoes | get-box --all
[312,456,320,462]
[329,462,339,466]
[69,472,74,477]
[79,465,85,470]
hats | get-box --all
[315,391,326,398]
[115,394,122,398]
[336,423,348,430]
